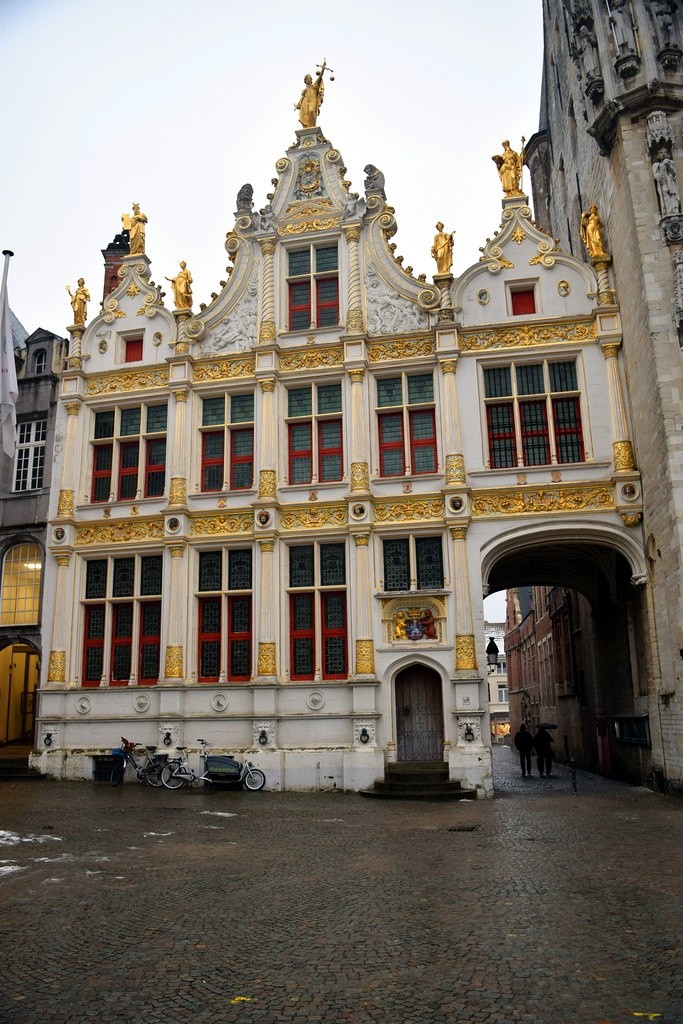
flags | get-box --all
[0,287,18,458]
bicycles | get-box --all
[108,736,173,787]
[161,738,266,793]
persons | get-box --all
[66,278,90,327]
[652,146,681,219]
[648,0,678,52]
[580,203,608,257]
[514,723,534,777]
[533,728,553,776]
[121,202,148,254]
[293,61,326,128]
[577,25,603,88]
[165,261,193,309]
[608,0,637,60]
[491,140,526,198]
[431,222,454,275]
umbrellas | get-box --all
[535,722,558,729]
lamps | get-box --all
[486,637,499,665]
